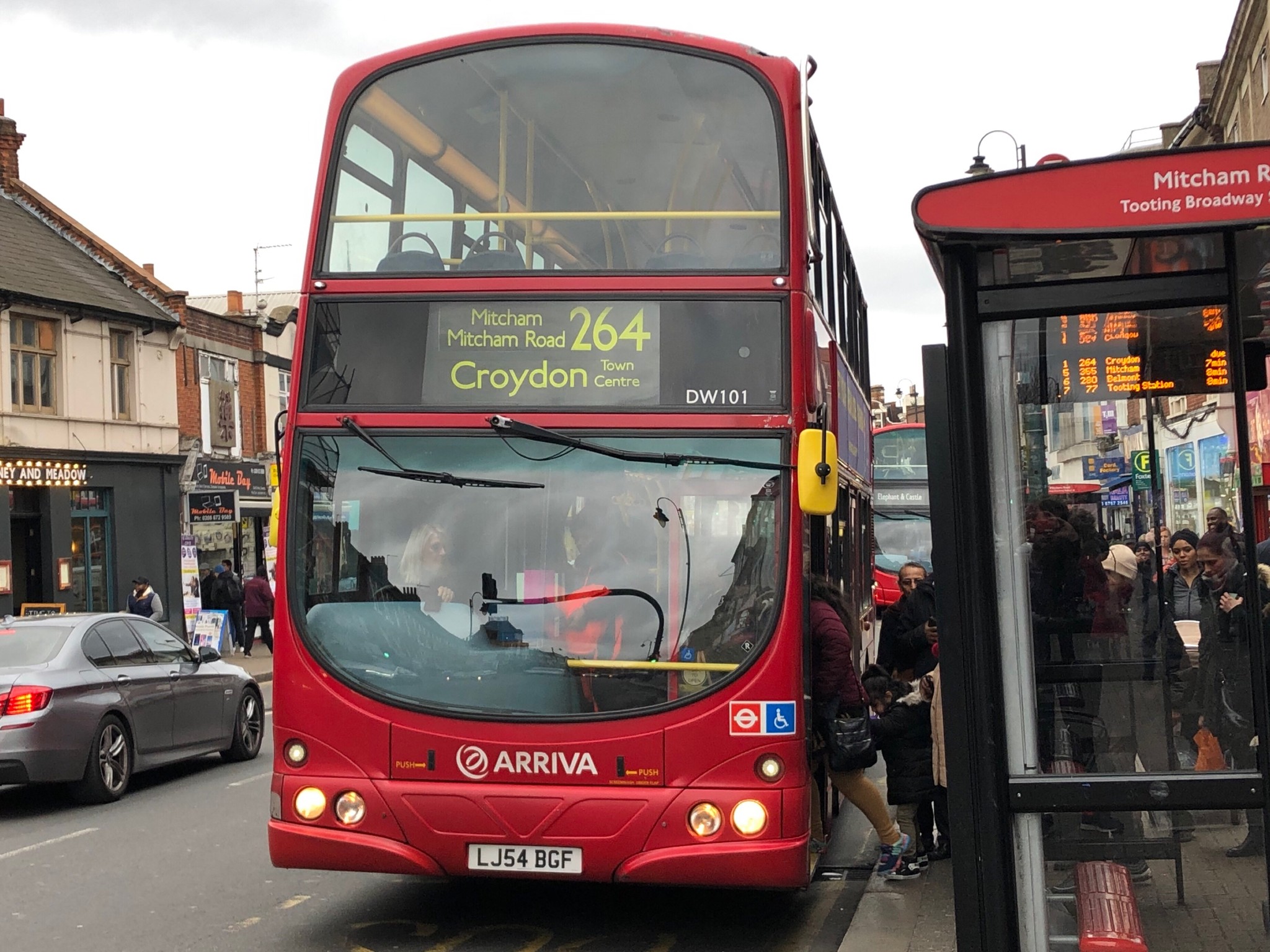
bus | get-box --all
[866,420,940,614]
[262,20,883,890]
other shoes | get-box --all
[233,646,236,653]
[239,647,244,652]
[1174,830,1192,842]
[244,651,252,658]
[877,832,951,880]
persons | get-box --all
[1023,496,1270,889]
[859,563,953,881]
[125,576,163,622]
[812,576,911,876]
[399,453,656,668]
[199,560,276,657]
[185,577,200,598]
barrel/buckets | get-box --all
[1174,620,1202,667]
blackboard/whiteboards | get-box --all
[21,603,66,615]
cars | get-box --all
[0,609,268,808]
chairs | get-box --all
[375,232,444,271]
[642,233,717,268]
[726,232,780,269]
[458,232,526,271]
[884,468,905,478]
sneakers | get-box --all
[1080,811,1121,832]
[809,838,827,854]
[1112,858,1151,879]
[1051,873,1075,893]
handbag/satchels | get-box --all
[544,584,622,663]
[824,718,877,772]
[1193,727,1227,771]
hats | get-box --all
[199,563,209,569]
[1101,544,1138,581]
[1122,533,1135,544]
[214,565,225,574]
[132,576,149,585]
[1145,528,1154,543]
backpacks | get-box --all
[218,575,245,604]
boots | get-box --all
[1226,827,1264,857]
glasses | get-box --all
[903,577,924,585]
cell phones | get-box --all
[928,616,938,632]
